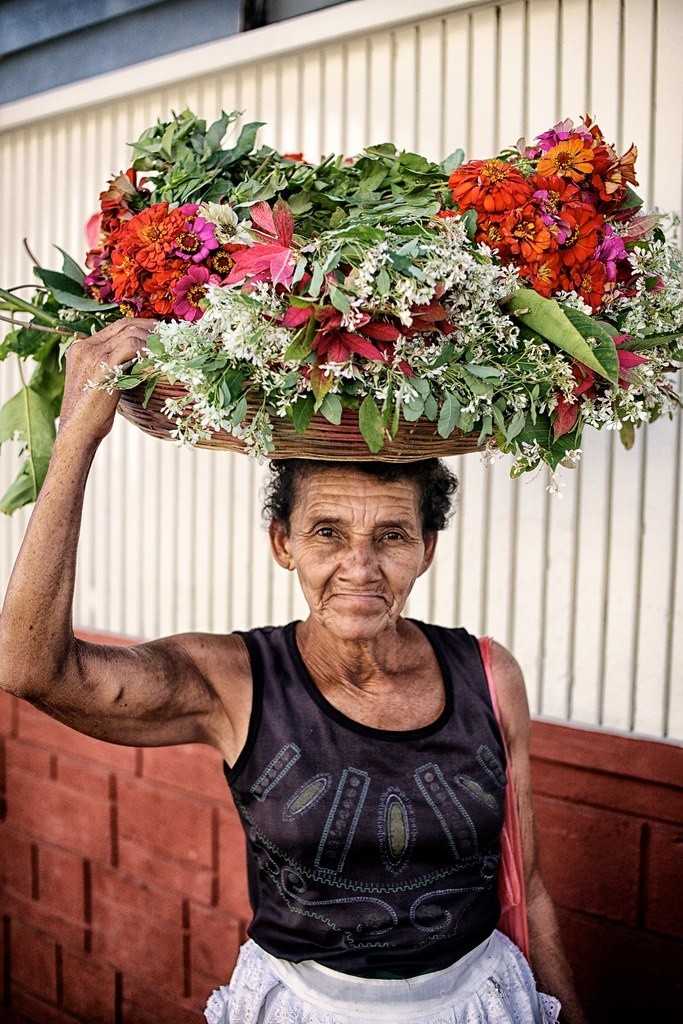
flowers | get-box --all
[0,102,683,517]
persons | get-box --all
[0,318,587,1024]
